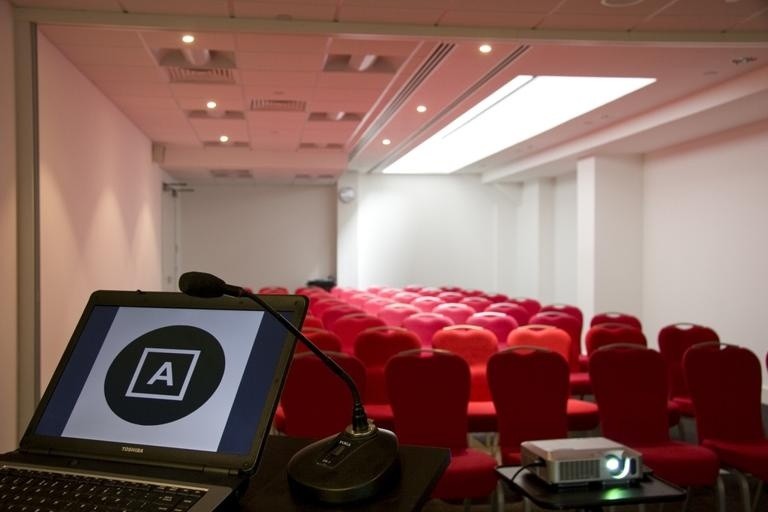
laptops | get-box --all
[1,290,307,511]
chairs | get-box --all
[242,284,767,512]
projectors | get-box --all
[521,437,641,488]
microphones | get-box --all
[179,272,400,492]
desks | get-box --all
[493,463,687,512]
[236,435,452,512]
